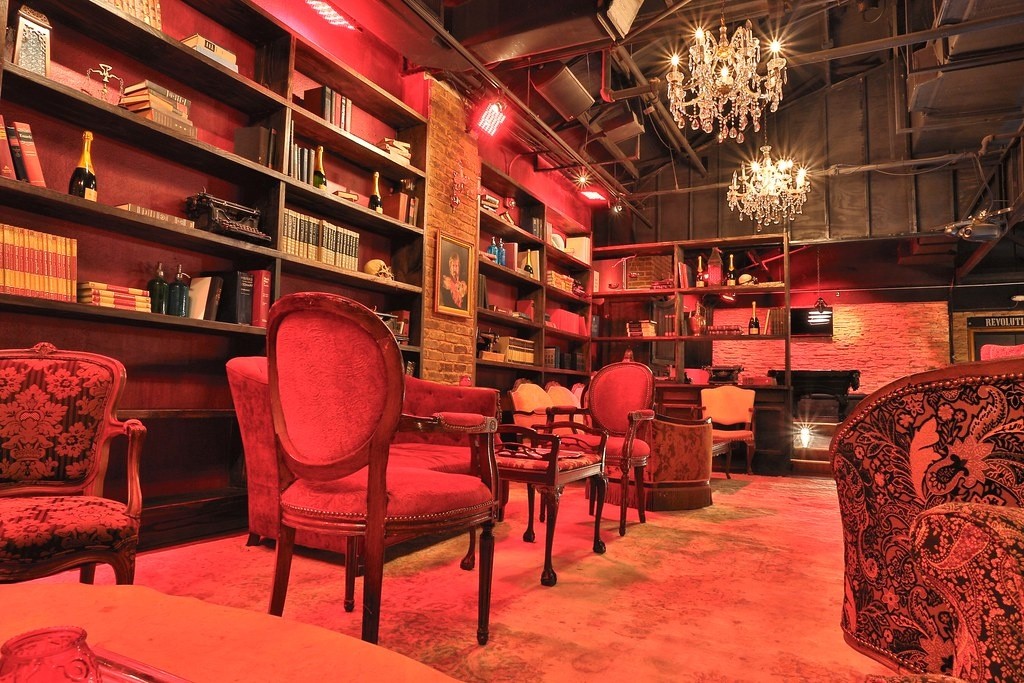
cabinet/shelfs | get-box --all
[0,0,428,551]
[473,161,594,414]
[592,230,794,476]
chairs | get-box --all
[0,291,756,683]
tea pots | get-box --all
[684,300,707,336]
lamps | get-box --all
[814,248,828,312]
[580,189,607,200]
[726,83,812,232]
[666,0,787,143]
[307,0,363,31]
[467,99,508,141]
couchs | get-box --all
[828,357,1024,683]
[225,354,510,566]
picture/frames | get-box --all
[434,229,474,318]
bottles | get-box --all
[696,256,704,288]
[487,236,506,266]
[168,264,190,317]
[748,301,760,335]
[368,171,383,214]
[313,145,326,190]
[524,249,533,279]
[727,254,735,286]
[147,261,169,314]
[68,131,98,202]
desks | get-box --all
[767,369,862,422]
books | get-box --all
[0,1,591,373]
[625,281,776,386]
